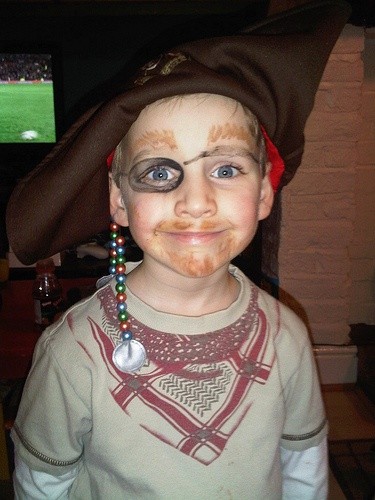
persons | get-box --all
[8,0,353,500]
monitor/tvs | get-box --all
[0,42,64,152]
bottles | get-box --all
[32,258,65,325]
